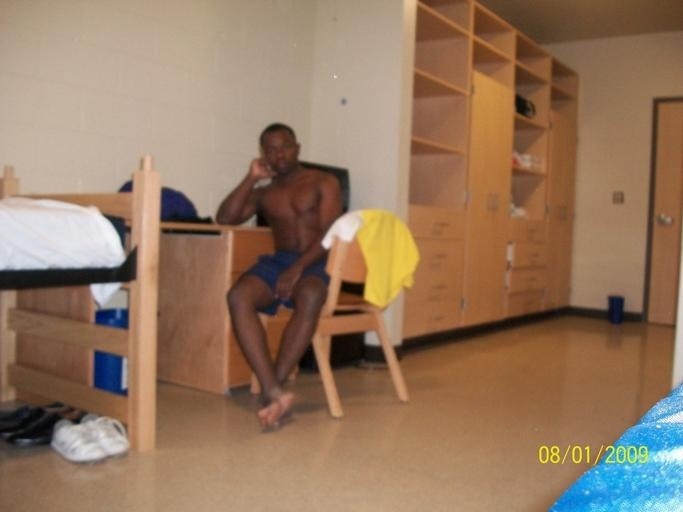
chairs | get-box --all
[248,207,414,418]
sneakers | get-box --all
[50,412,128,462]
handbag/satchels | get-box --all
[120,180,213,224]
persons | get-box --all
[215,124,344,431]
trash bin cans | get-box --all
[95,308,130,395]
[609,296,623,323]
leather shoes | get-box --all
[1,401,87,446]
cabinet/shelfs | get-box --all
[403,0,580,340]
[159,222,297,396]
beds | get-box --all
[0,153,162,460]
